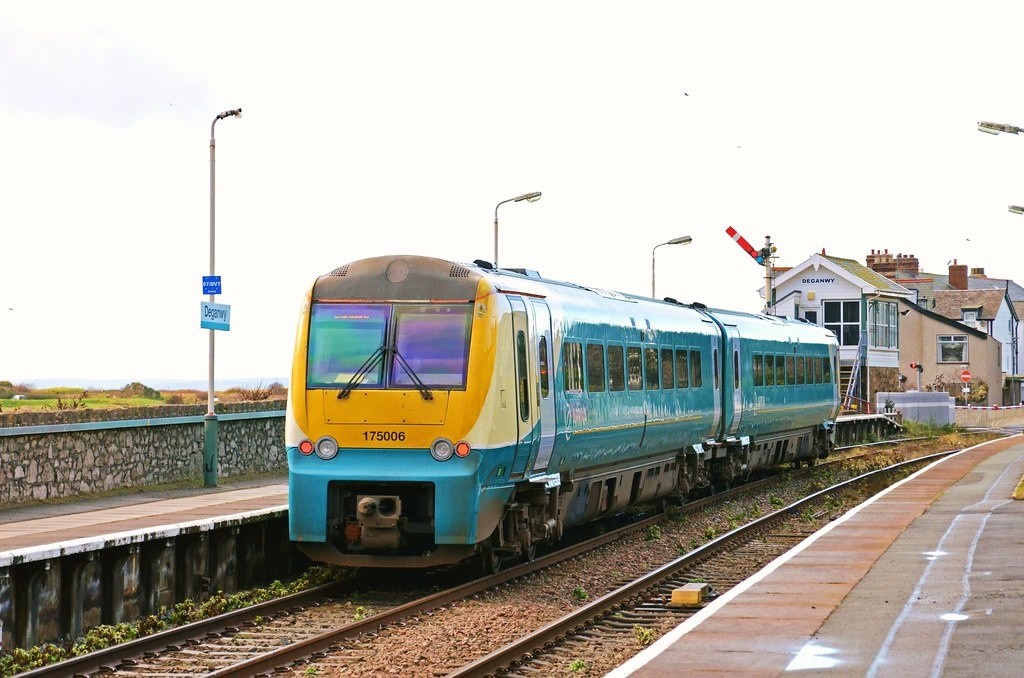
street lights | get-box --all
[494,191,542,267]
[652,235,692,298]
[203,107,243,488]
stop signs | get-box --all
[961,371,971,383]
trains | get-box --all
[284,253,842,576]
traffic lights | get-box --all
[910,363,917,369]
